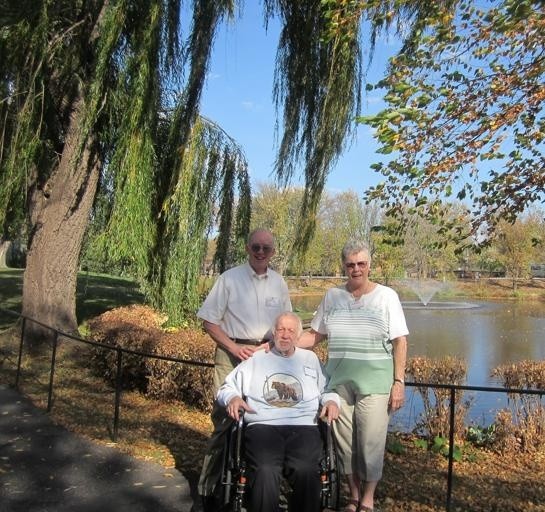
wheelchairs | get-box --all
[219,407,340,512]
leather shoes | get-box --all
[193,495,210,511]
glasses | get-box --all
[252,245,272,253]
[346,262,367,268]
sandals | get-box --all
[343,500,372,512]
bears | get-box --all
[271,381,298,401]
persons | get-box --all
[196,230,290,512]
[217,315,341,512]
[254,241,410,511]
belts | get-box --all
[229,338,269,346]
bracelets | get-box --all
[395,377,405,384]
